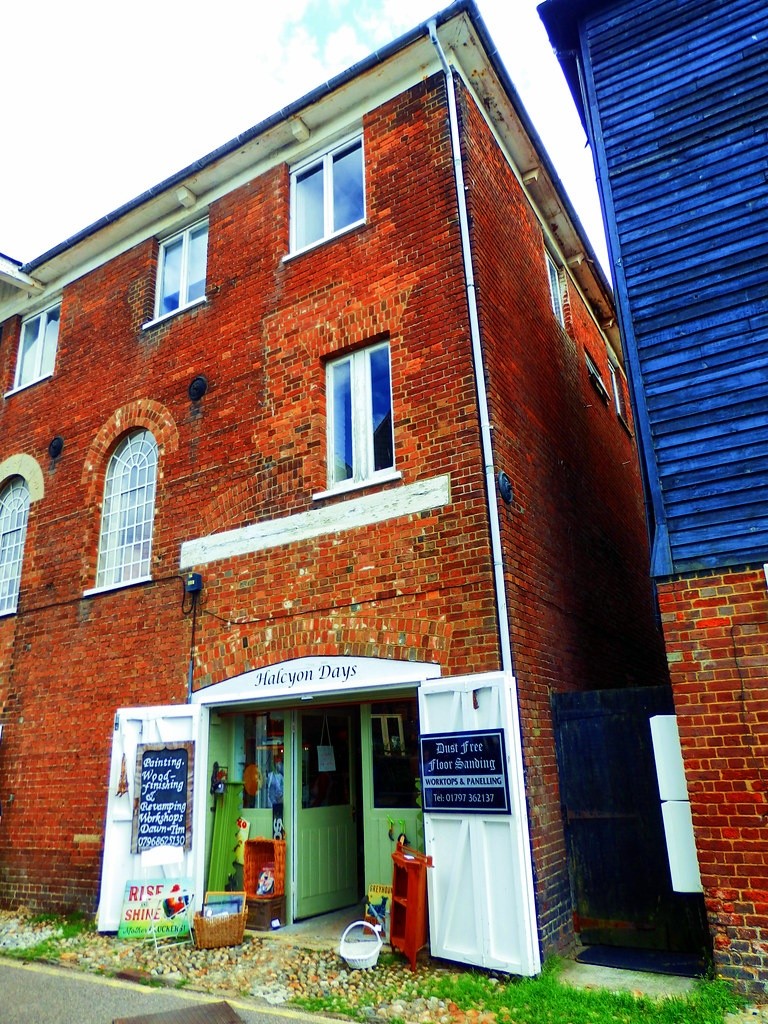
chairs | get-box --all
[141,845,194,953]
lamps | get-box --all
[211,761,225,794]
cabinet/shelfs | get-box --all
[391,842,435,973]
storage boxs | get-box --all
[246,895,287,930]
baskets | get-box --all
[193,906,249,949]
[338,921,382,969]
[243,838,287,899]
